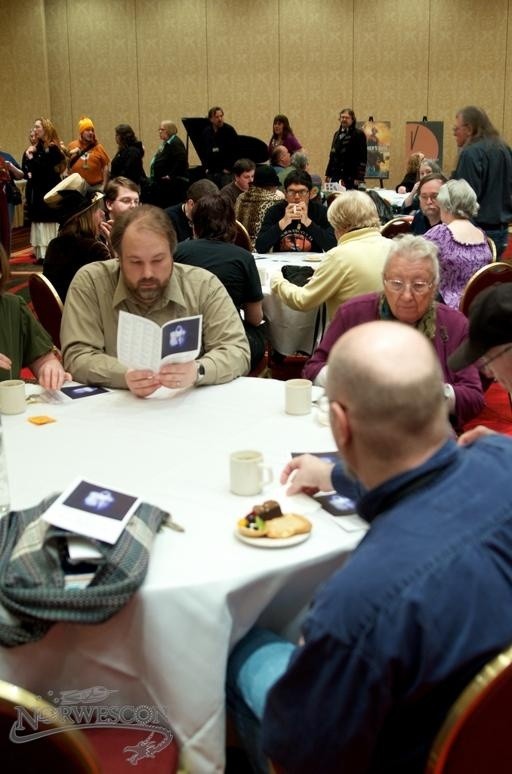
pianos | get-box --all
[180,117,269,185]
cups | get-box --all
[229,451,273,497]
[0,379,25,415]
[258,266,269,285]
[285,378,312,415]
[292,204,301,219]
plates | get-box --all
[303,258,322,262]
[235,531,311,549]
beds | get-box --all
[246,250,331,375]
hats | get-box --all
[79,115,94,133]
[447,281,512,372]
[44,173,106,231]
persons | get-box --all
[0,105,511,449]
[231,319,511,774]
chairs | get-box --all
[380,218,415,237]
[27,272,64,354]
[0,679,107,773]
[235,218,255,252]
[459,261,512,366]
[424,645,512,774]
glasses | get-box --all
[476,346,512,369]
[383,279,432,295]
[287,190,309,196]
